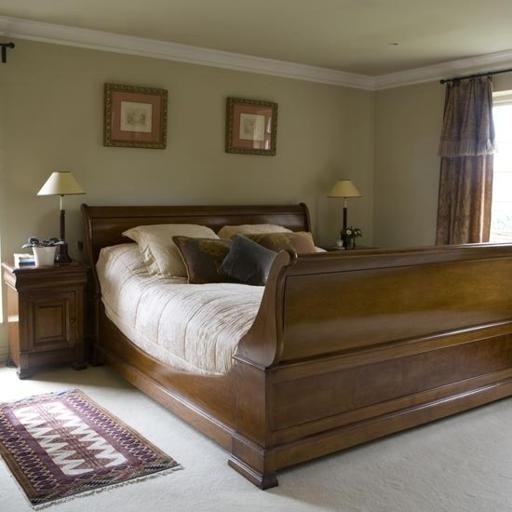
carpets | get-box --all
[0,386,185,511]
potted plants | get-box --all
[24,236,65,265]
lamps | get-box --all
[37,171,87,265]
[327,180,362,249]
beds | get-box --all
[82,203,512,490]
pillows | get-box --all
[122,224,315,285]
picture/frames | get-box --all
[101,82,170,150]
[223,96,279,156]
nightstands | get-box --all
[1,257,89,379]
